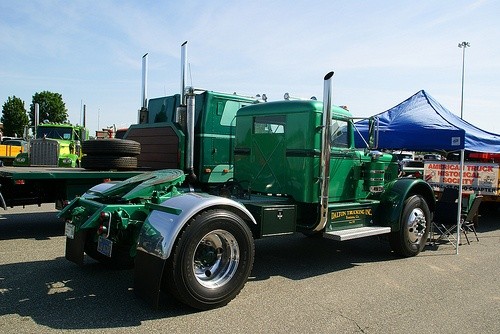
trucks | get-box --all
[0,39,261,209]
[56,69,435,309]
[10,103,89,168]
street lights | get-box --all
[458,41,472,120]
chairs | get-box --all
[431,194,485,253]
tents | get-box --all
[332,90,500,255]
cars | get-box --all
[2,139,24,146]
